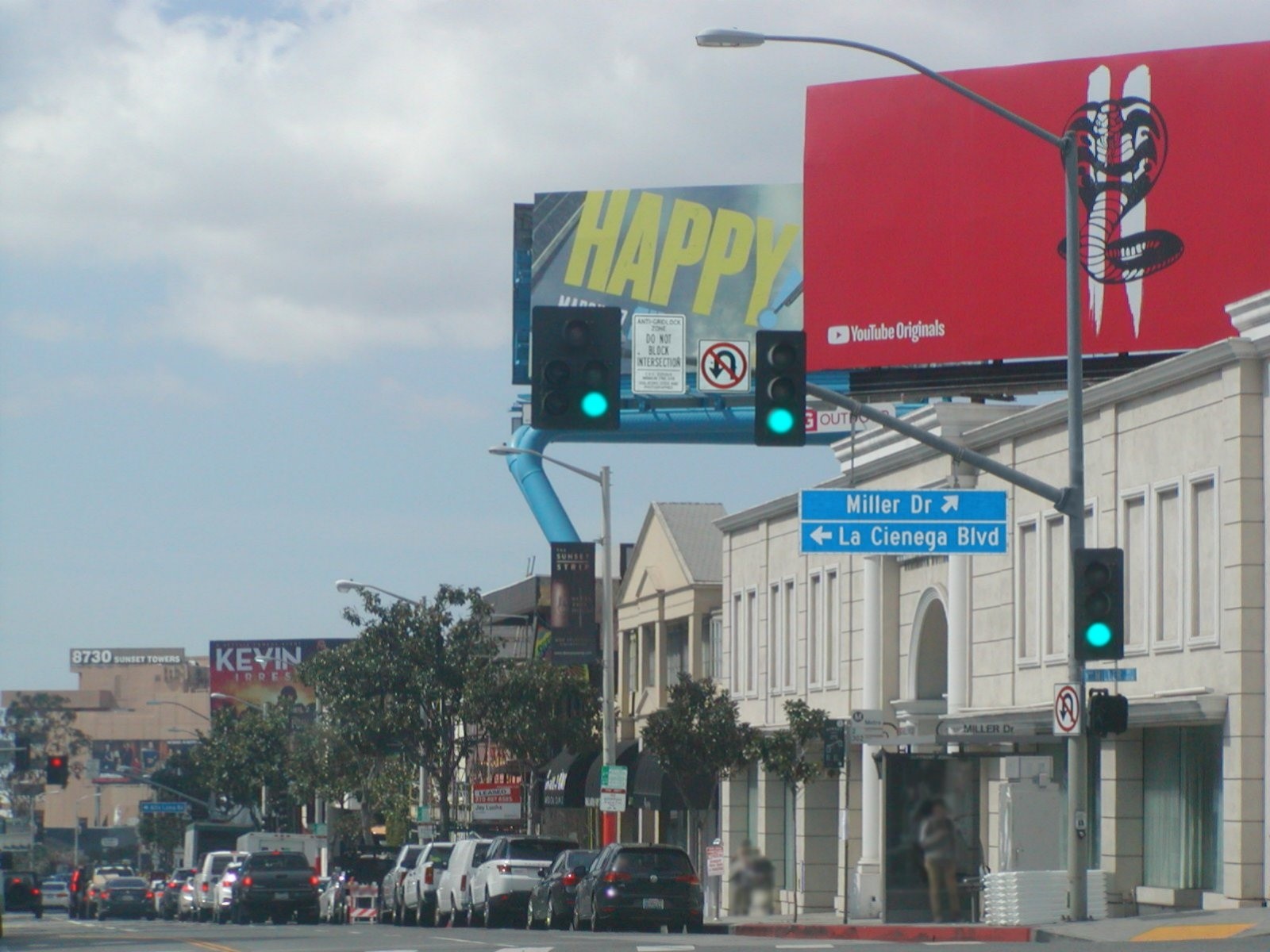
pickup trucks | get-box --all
[227,850,322,926]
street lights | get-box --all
[334,579,429,846]
[694,28,1091,922]
[488,441,619,847]
[209,691,272,817]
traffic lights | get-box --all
[46,755,68,785]
[1073,548,1126,662]
[1089,693,1128,735]
[755,330,808,447]
[532,305,621,430]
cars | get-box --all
[526,849,605,931]
[40,881,72,913]
[49,848,252,925]
[571,842,705,934]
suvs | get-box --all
[466,833,580,928]
[0,869,46,919]
[318,838,494,929]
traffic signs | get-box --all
[797,488,1008,523]
[796,519,1008,553]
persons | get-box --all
[732,840,754,916]
[920,798,964,925]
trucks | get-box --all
[235,831,319,872]
